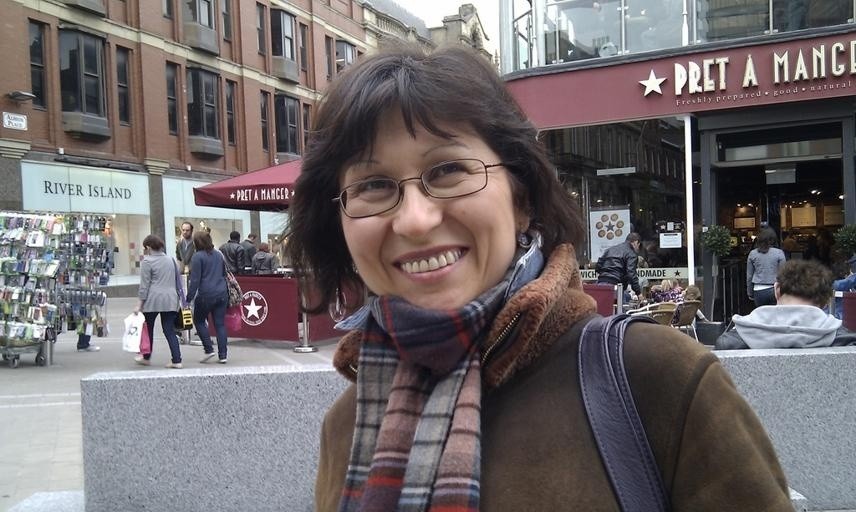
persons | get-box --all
[638,244,683,268]
[783,227,799,251]
[715,255,856,350]
[745,228,786,307]
[251,242,276,275]
[185,230,232,364]
[595,232,644,312]
[133,234,185,368]
[218,231,245,276]
[816,229,830,261]
[271,44,794,512]
[241,233,258,275]
[175,221,195,274]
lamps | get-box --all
[4,90,36,101]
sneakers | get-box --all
[78,345,100,352]
[134,355,150,365]
[165,362,182,369]
[218,358,228,364]
[199,352,216,362]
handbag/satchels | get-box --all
[120,311,152,356]
[222,311,244,332]
[174,306,194,331]
[224,273,245,308]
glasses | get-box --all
[330,159,507,219]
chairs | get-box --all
[626,283,702,343]
